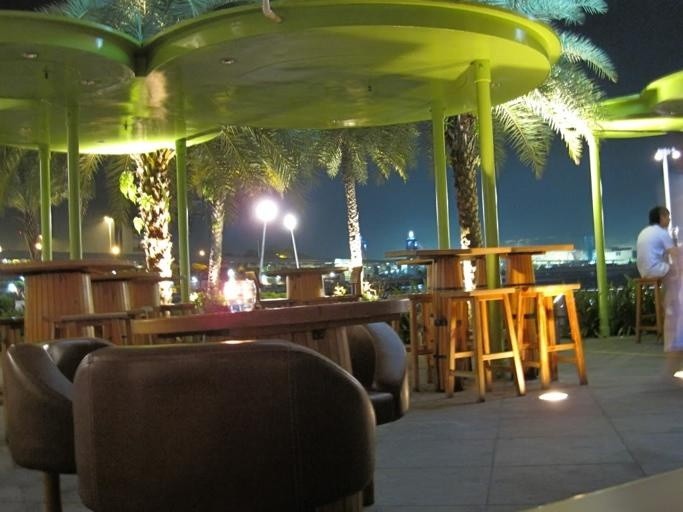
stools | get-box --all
[633,276,665,343]
[408,281,589,402]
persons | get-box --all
[637,207,679,280]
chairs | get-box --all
[72,340,376,511]
[245,266,363,303]
[41,302,195,345]
[5,343,76,511]
[50,342,120,380]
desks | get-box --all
[385,244,575,392]
[130,296,412,425]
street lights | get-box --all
[282,213,299,268]
[254,199,277,271]
[653,146,680,265]
[103,216,114,252]
